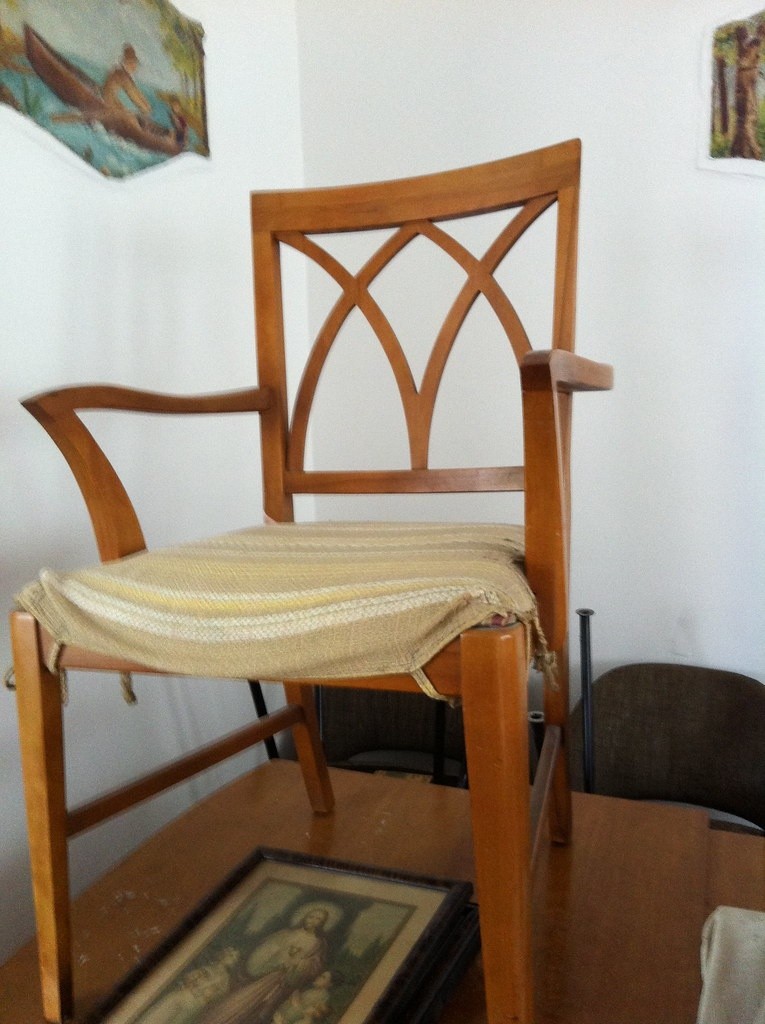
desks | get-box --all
[0,761,765,1024]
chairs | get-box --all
[315,685,469,790]
[562,662,765,837]
[0,136,614,1024]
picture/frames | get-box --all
[384,901,480,1024]
[79,842,475,1024]
